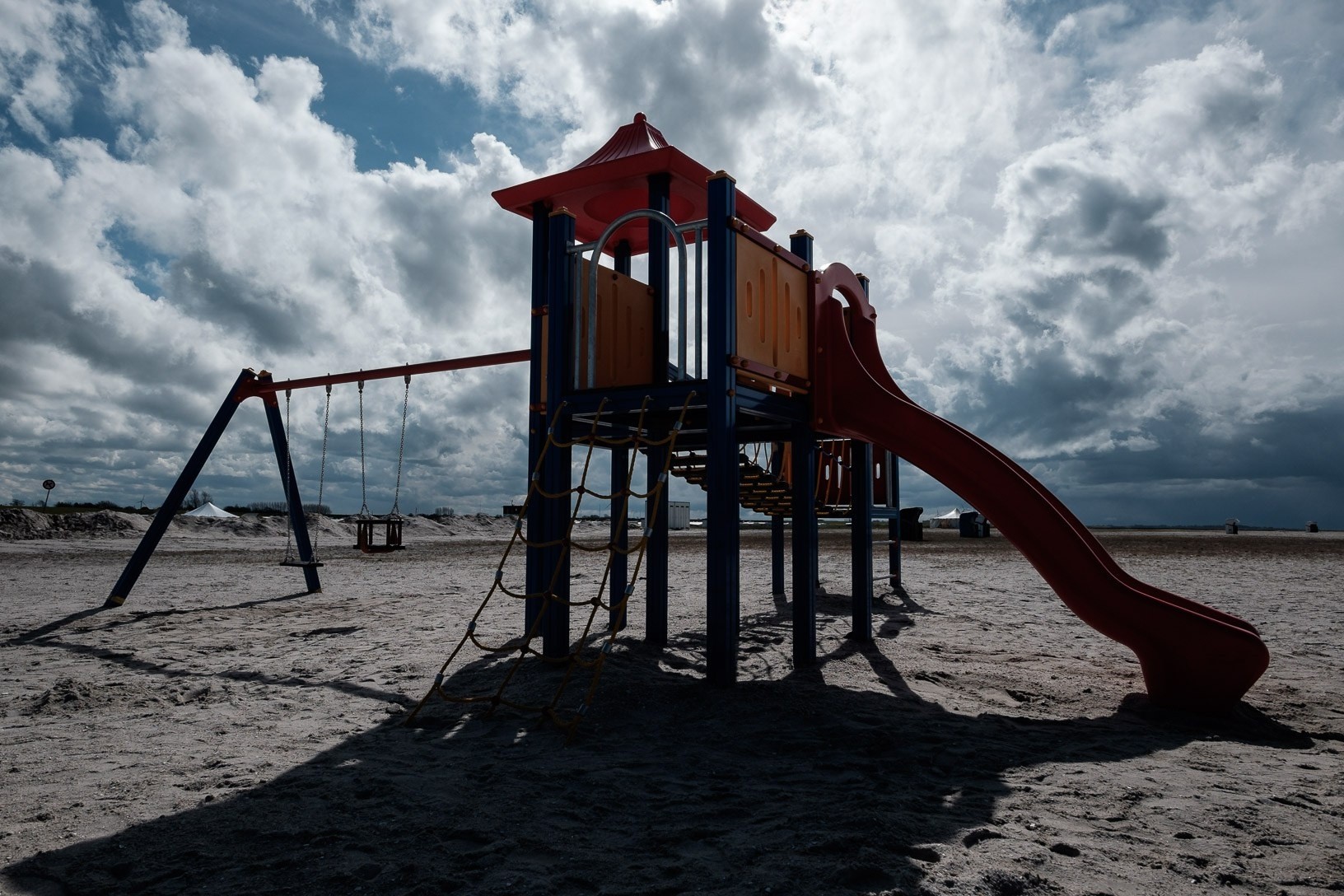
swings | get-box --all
[352,373,411,554]
[279,383,332,567]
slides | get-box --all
[822,304,1271,708]
